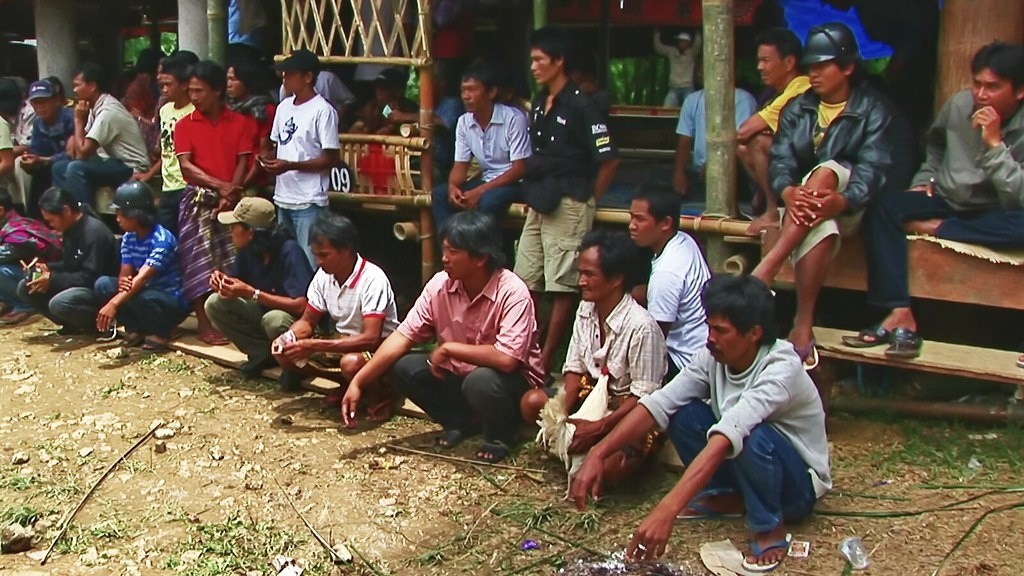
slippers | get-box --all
[743,533,792,570]
[676,493,742,519]
[842,323,892,348]
[787,336,819,370]
[885,327,922,357]
[142,332,182,352]
[435,429,465,449]
[120,332,144,347]
[196,330,229,345]
[476,440,511,463]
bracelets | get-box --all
[137,115,141,122]
[243,288,260,305]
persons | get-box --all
[750,22,896,371]
[519,226,669,489]
[571,273,833,571]
[1,1,758,464]
[842,40,1024,368]
[737,26,811,237]
[629,183,712,382]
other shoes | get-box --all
[95,317,116,342]
[279,368,306,392]
[240,351,280,377]
[366,393,392,421]
[0,310,41,328]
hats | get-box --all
[217,196,275,230]
[26,80,57,100]
[274,50,321,74]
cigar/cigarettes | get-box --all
[425,359,433,367]
[259,161,266,169]
[636,542,648,553]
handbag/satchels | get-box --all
[524,155,560,214]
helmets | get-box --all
[798,22,858,65]
[109,180,154,208]
[676,31,691,42]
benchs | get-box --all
[806,325,1024,434]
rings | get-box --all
[96,318,98,322]
